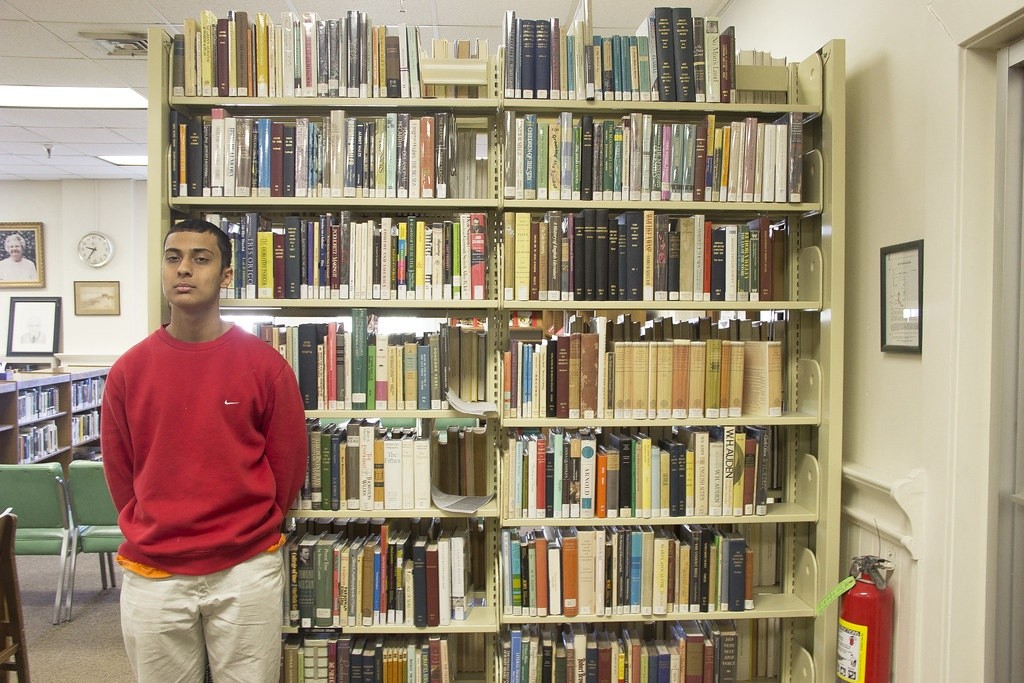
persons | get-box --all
[0,235,37,282]
[100,219,308,683]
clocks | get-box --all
[78,231,115,268]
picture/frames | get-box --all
[879,239,923,353]
[6,297,61,357]
[0,221,45,290]
[74,280,121,315]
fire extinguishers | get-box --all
[834,557,895,683]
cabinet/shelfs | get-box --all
[145,1,846,683]
[0,366,111,482]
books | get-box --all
[169,1,806,683]
[18,378,106,465]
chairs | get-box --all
[0,461,108,626]
[64,457,128,623]
[1,507,32,683]
